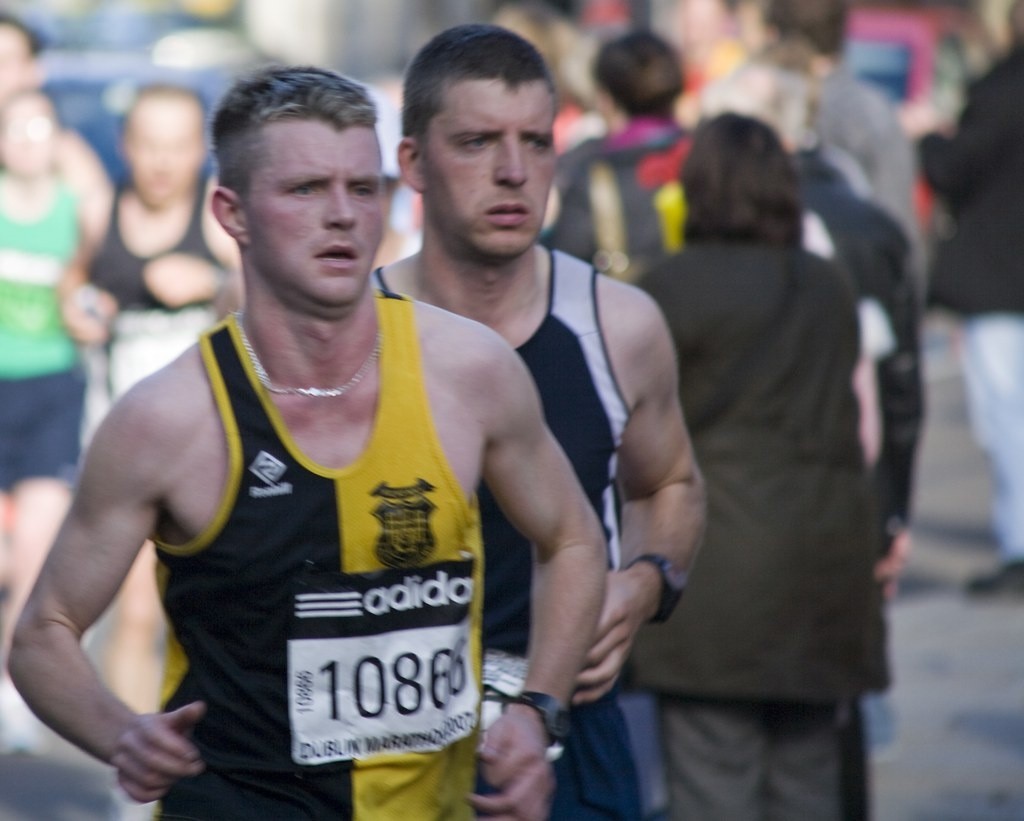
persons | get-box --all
[370,24,705,821]
[622,114,891,820]
[0,1,1023,658]
[10,67,611,821]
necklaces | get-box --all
[235,306,379,396]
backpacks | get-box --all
[537,124,689,282]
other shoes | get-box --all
[967,556,1024,596]
[0,676,43,759]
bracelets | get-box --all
[626,554,682,624]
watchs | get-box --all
[511,691,571,738]
